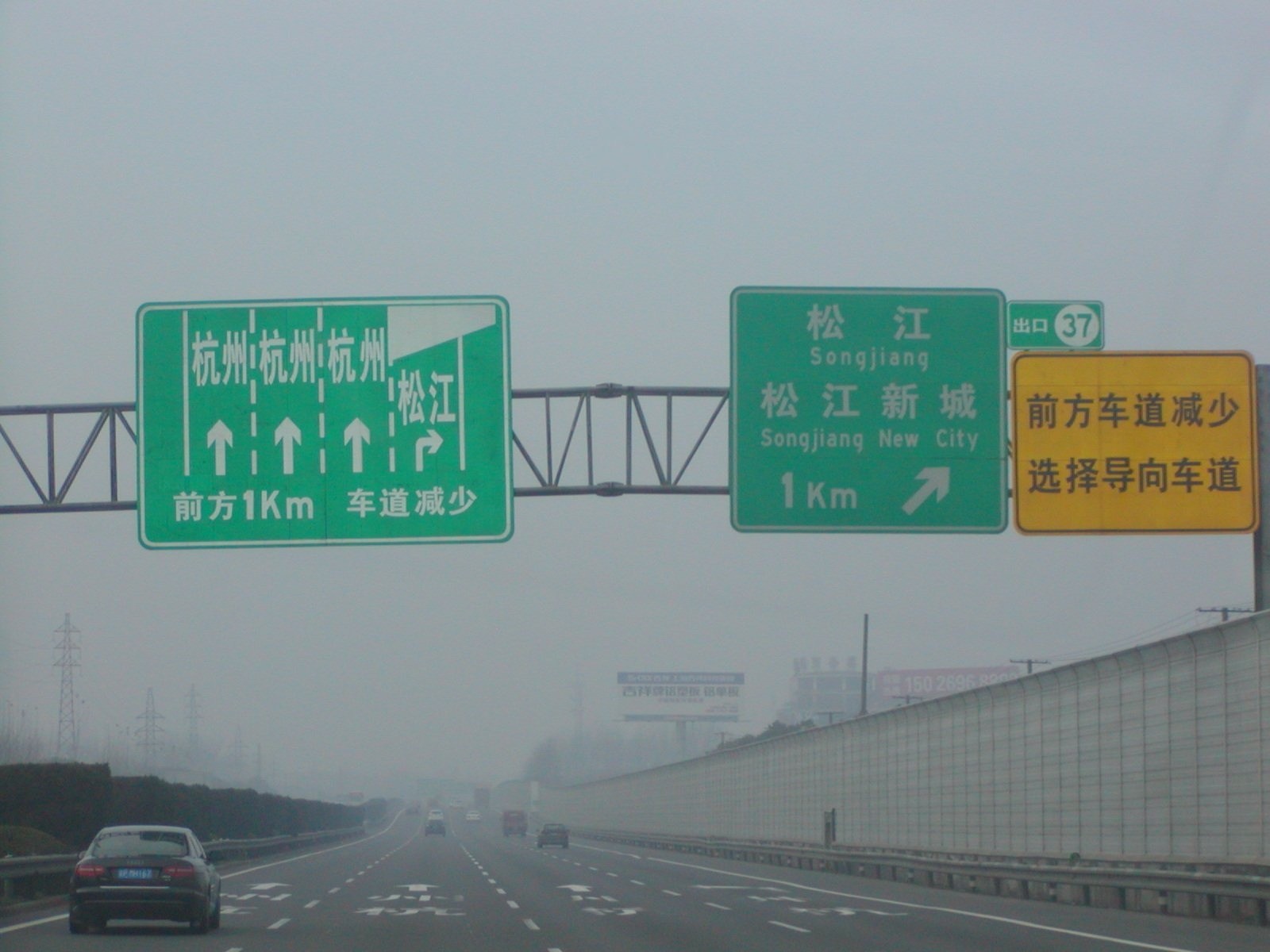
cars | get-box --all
[535,822,571,849]
[68,823,223,938]
[500,809,528,837]
[465,809,483,823]
[423,809,446,837]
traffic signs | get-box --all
[134,294,515,551]
[727,283,1010,534]
